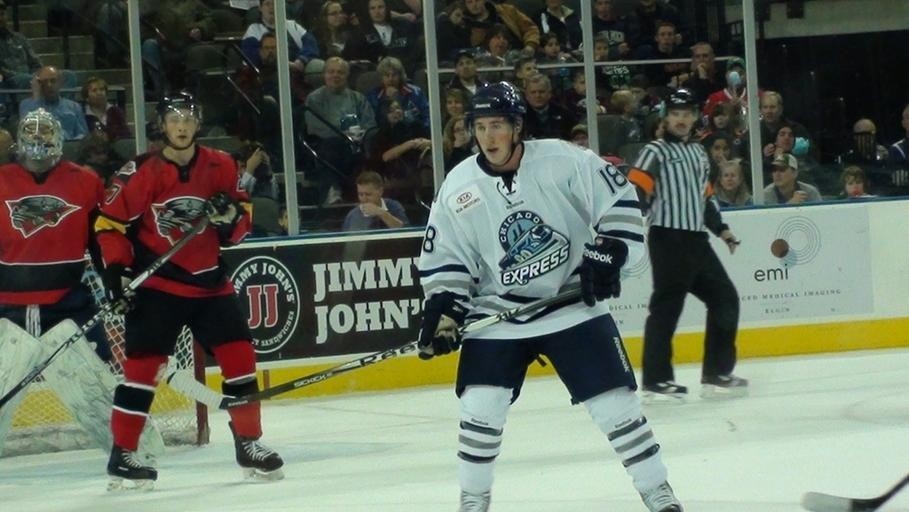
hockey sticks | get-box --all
[803,474,907,511]
[167,286,589,411]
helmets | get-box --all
[468,79,531,131]
[155,88,205,128]
[664,86,700,117]
[13,107,66,168]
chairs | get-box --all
[0,0,431,238]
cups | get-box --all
[360,204,369,217]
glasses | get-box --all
[771,167,793,173]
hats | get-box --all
[770,153,798,170]
[726,56,745,72]
[454,47,476,64]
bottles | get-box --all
[349,125,361,137]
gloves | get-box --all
[579,236,629,307]
[102,261,138,315]
[203,190,246,234]
[415,295,469,360]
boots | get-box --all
[228,420,284,471]
[638,479,683,512]
[105,441,158,481]
[699,364,749,389]
[641,377,688,397]
[457,488,491,512]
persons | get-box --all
[629,88,748,394]
[0,109,163,464]
[416,81,683,512]
[95,93,284,482]
